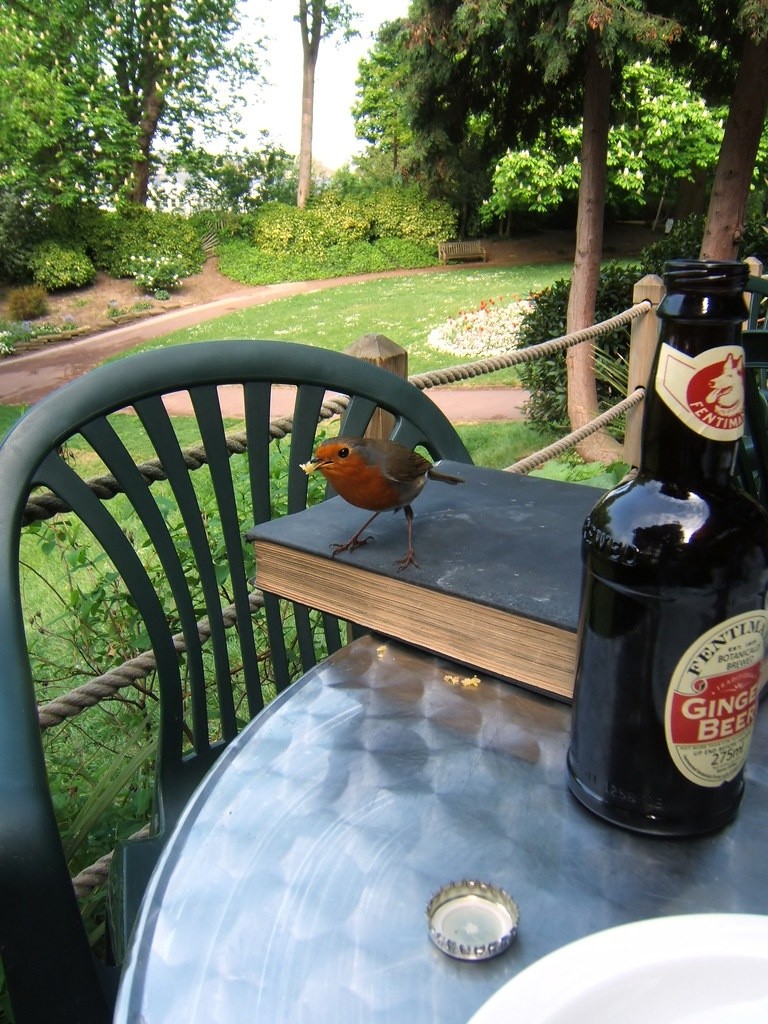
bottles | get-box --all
[567,259,768,835]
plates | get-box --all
[469,913,768,1024]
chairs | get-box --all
[0,338,477,1024]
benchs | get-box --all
[437,240,488,266]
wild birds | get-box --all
[299,436,466,572]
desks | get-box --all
[115,634,768,1024]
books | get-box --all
[244,457,613,708]
